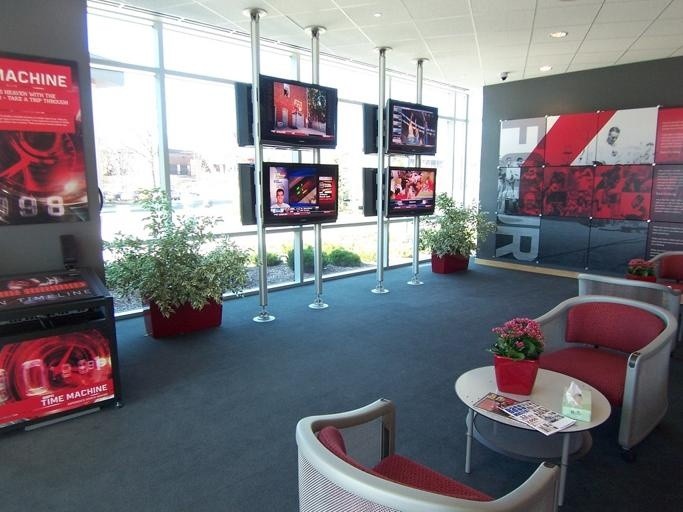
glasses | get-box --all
[610,135,618,139]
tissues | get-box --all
[561,380,592,422]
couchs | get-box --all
[577,273,679,319]
[648,249,683,292]
[528,294,678,458]
[297,397,558,512]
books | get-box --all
[501,399,577,436]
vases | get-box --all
[494,354,539,395]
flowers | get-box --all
[484,316,546,360]
[627,258,654,276]
[626,275,657,283]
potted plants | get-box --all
[418,189,498,275]
[106,186,256,340]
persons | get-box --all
[270,189,290,213]
[593,127,621,166]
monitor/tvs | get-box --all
[386,96,440,155]
[258,74,338,149]
[263,162,339,227]
[382,166,436,218]
